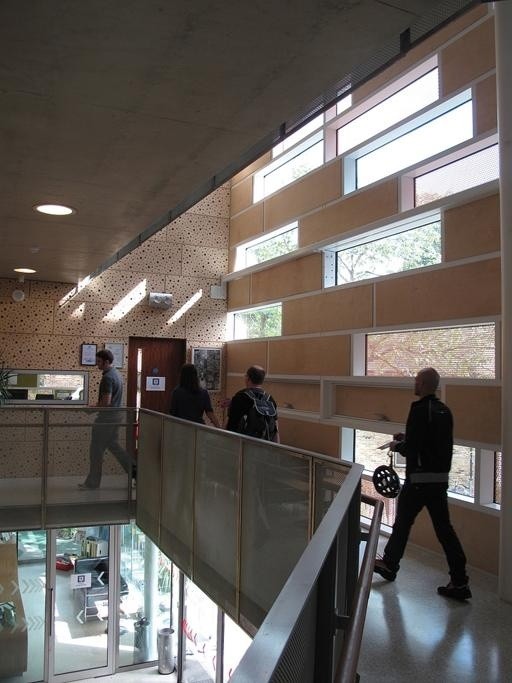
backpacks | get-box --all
[237,389,279,441]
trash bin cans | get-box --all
[134,618,150,662]
[157,628,175,675]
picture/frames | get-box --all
[191,347,223,394]
[81,343,97,366]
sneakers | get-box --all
[374,555,400,581]
[437,575,472,600]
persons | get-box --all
[78,349,138,488]
[171,361,221,431]
[223,364,282,546]
[371,367,474,599]
